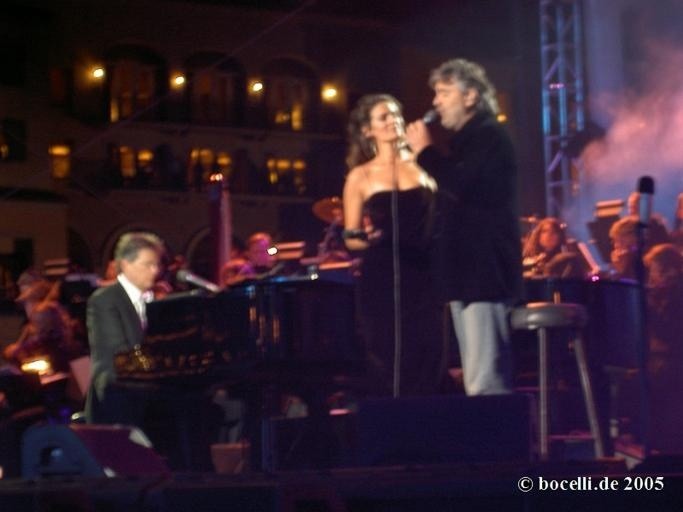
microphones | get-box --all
[401,110,436,150]
[637,177,656,241]
[175,269,217,291]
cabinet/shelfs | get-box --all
[70,104,356,211]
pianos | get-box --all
[106,263,649,471]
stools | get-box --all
[508,298,610,471]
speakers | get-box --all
[19,422,172,483]
[357,394,539,467]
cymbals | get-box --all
[313,196,344,226]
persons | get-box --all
[0,193,683,463]
[342,94,448,395]
[403,57,522,397]
